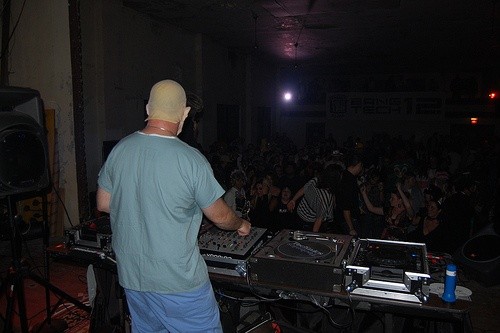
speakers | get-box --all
[0,112,49,191]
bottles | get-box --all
[441,263,457,302]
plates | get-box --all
[430,282,472,297]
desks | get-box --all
[48,226,474,333]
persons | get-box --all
[211,127,500,255]
[96,79,250,333]
